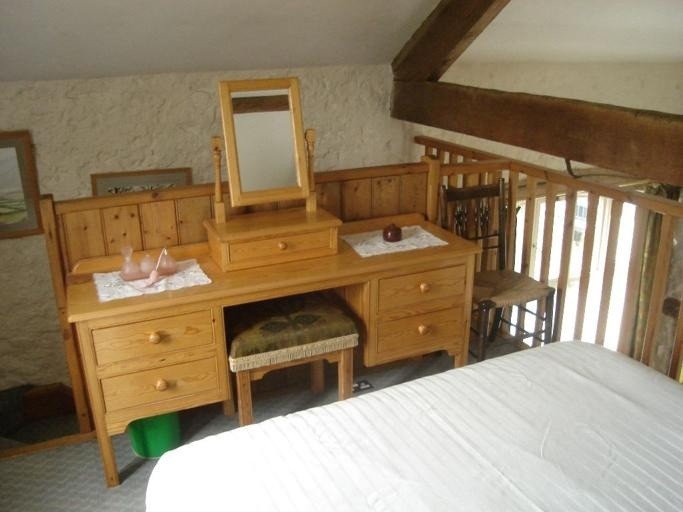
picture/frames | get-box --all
[0,129,45,241]
[89,166,193,198]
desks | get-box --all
[64,212,485,489]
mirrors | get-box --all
[216,76,311,208]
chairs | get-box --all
[437,178,556,364]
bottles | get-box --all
[382,224,401,242]
[120,244,176,283]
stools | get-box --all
[226,292,361,427]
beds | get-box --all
[143,337,681,512]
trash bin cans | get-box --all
[128,411,182,459]
